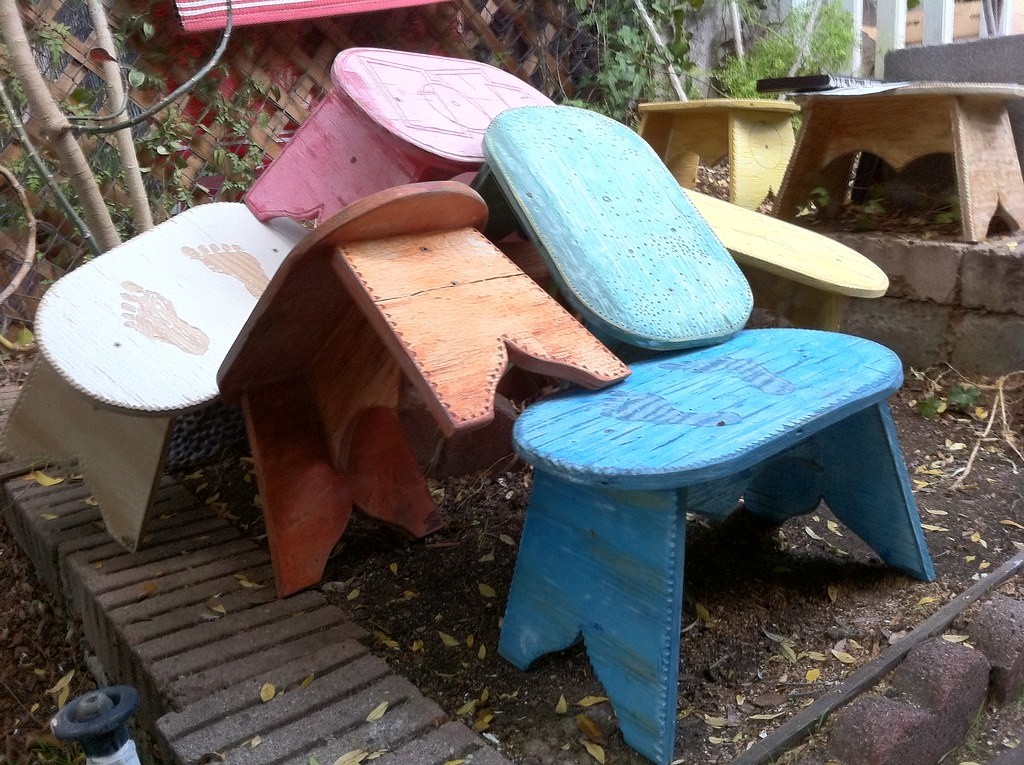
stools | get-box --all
[0,46,1024,765]
